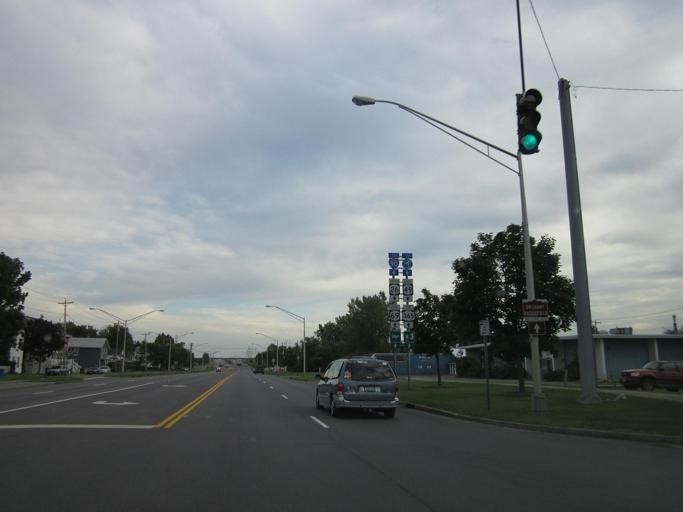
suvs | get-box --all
[620,361,682,392]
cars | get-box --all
[87,365,111,374]
[252,365,264,375]
[315,356,399,418]
[216,367,222,372]
[46,365,69,376]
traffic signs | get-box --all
[522,300,548,317]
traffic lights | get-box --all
[516,89,543,155]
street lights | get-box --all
[168,332,193,369]
[251,343,268,367]
[89,307,164,372]
[353,96,543,398]
[256,332,278,371]
[266,305,305,374]
[190,342,208,370]
